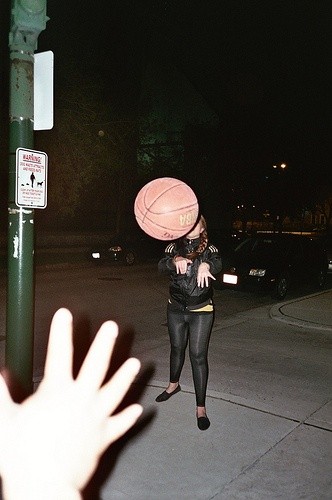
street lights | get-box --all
[272,163,287,168]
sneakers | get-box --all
[156,384,181,402]
[197,407,210,430]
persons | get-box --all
[0,307,143,500]
[156,213,223,431]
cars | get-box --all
[220,232,329,300]
[91,230,165,266]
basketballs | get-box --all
[134,178,199,240]
